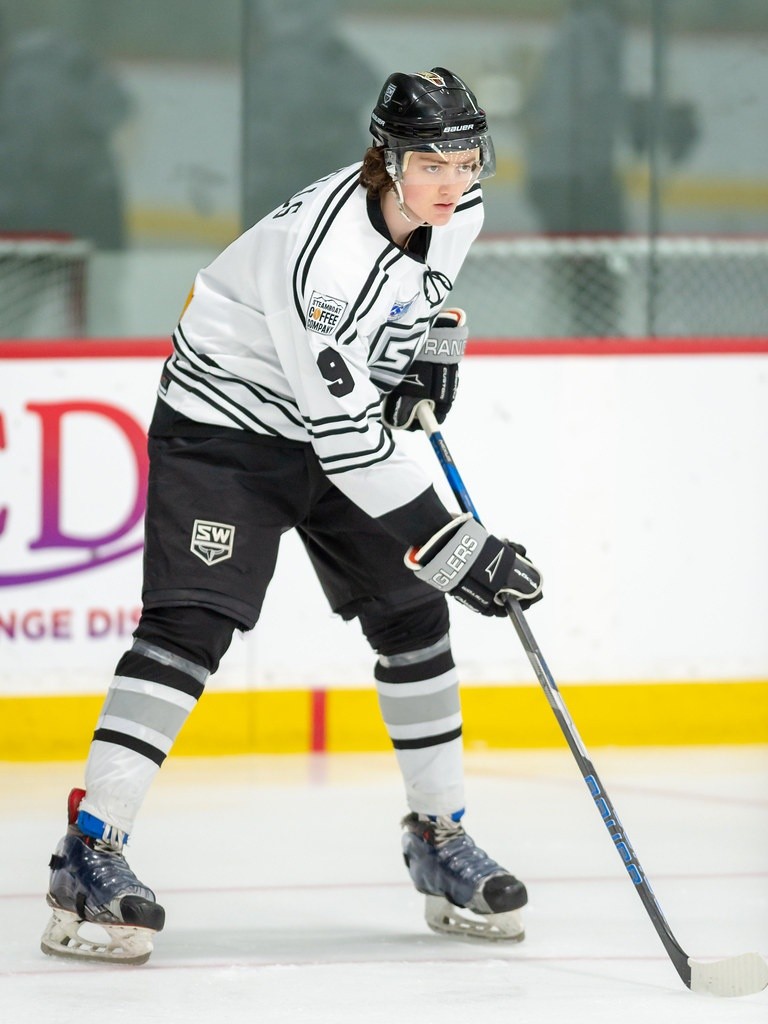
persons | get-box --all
[37,65,547,964]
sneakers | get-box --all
[40,822,166,964]
[400,812,530,942]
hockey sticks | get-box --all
[414,403,768,998]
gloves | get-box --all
[401,512,545,618]
[378,307,468,432]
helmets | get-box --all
[367,66,489,153]
